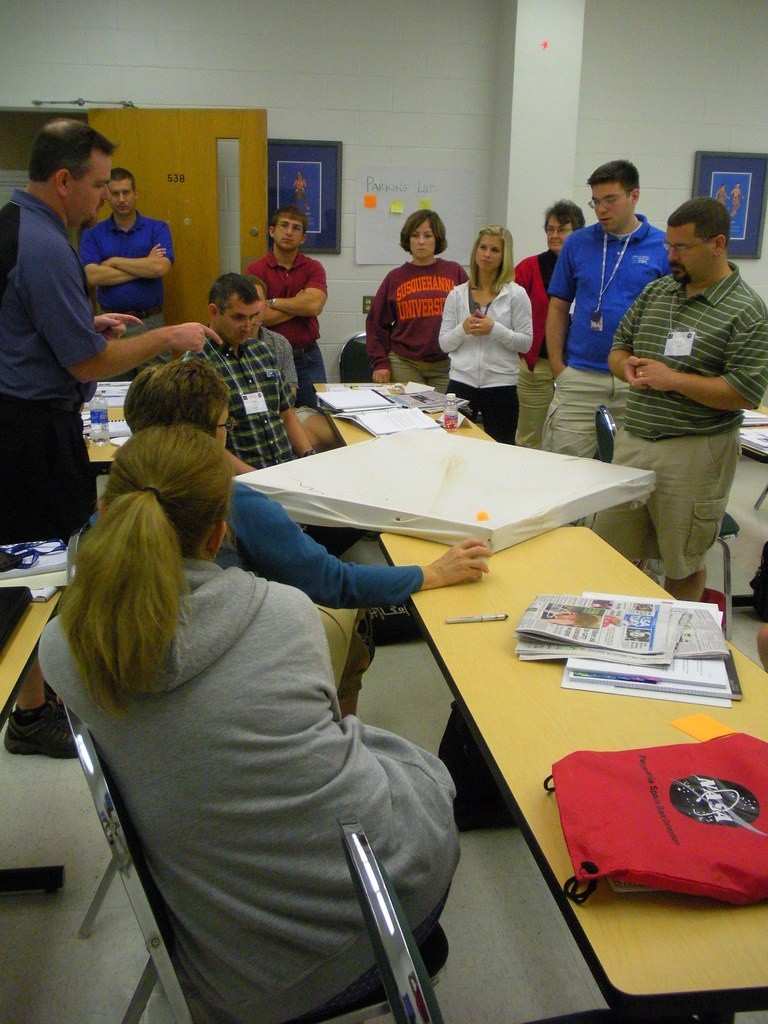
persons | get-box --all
[182,275,368,560]
[542,161,671,458]
[0,119,222,759]
[38,424,457,1024]
[516,203,586,449]
[247,276,378,542]
[123,357,492,717]
[81,167,174,376]
[246,207,327,408]
[366,209,468,395]
[590,195,767,602]
[440,225,532,447]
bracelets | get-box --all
[304,450,316,457]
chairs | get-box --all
[593,405,740,639]
[58,680,443,1024]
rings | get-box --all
[476,324,479,327]
[640,372,642,376]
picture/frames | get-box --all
[690,152,768,260]
[267,136,344,253]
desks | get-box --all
[77,380,136,471]
[0,502,101,896]
[314,382,768,1024]
[736,404,768,509]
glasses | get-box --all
[544,225,575,235]
[663,233,726,251]
[588,187,635,209]
[196,416,238,432]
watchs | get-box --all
[269,299,275,308]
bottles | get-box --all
[88,391,110,447]
[443,393,459,433]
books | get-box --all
[565,591,742,700]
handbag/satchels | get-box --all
[543,731,767,913]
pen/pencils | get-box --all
[269,371,277,384]
[349,385,360,391]
[445,614,509,625]
[571,671,646,681]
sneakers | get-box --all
[15,699,70,725]
[4,712,79,757]
